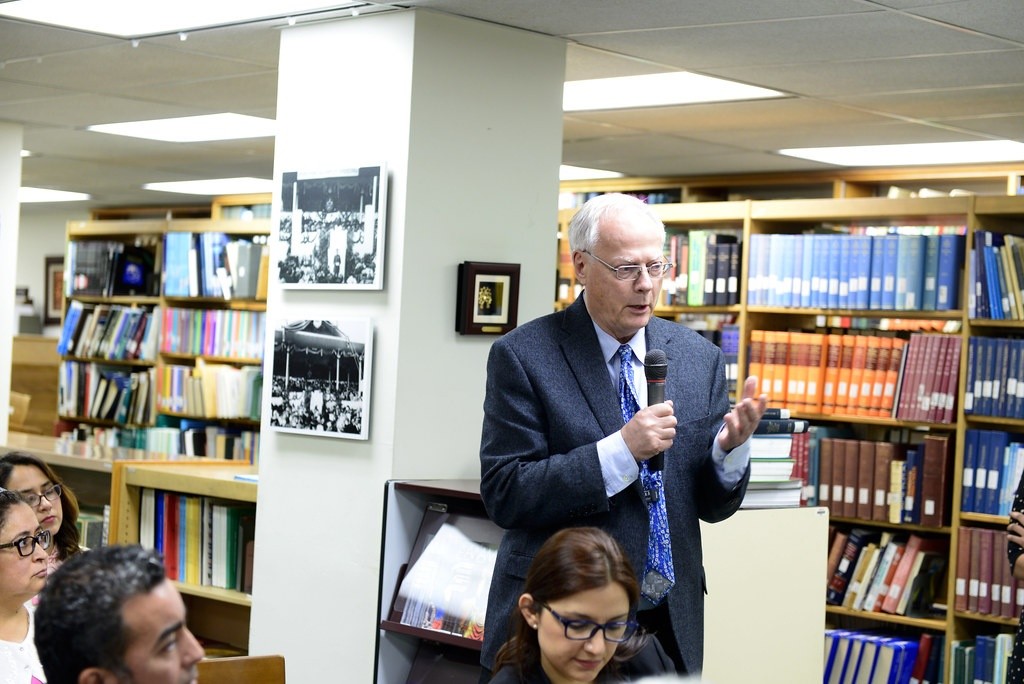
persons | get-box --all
[333,248,340,278]
[0,487,55,684]
[1007,468,1024,684]
[0,451,82,611]
[35,541,206,684]
[480,191,767,684]
[488,526,679,684]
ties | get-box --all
[616,344,676,608]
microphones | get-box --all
[643,348,667,470]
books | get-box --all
[56,232,268,467]
[556,192,1024,684]
[102,499,109,547]
[235,473,259,483]
[137,486,257,596]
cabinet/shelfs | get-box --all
[0,160,1024,684]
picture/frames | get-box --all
[455,261,522,335]
[45,256,65,324]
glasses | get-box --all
[585,251,674,281]
[538,604,639,643]
[23,483,61,508]
[0,530,51,557]
